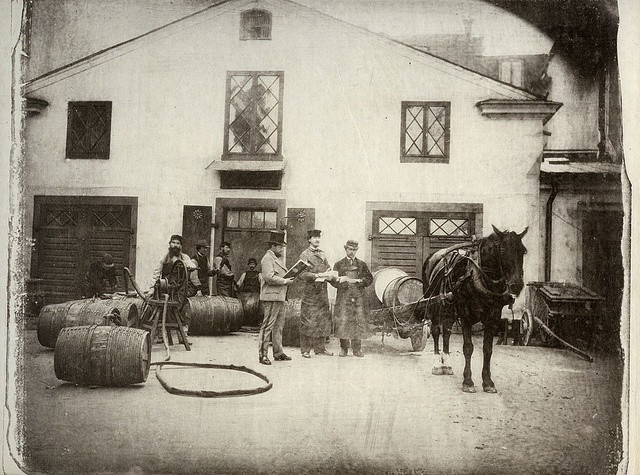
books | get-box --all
[314,271,338,282]
[282,259,312,280]
[339,275,362,284]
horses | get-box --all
[422,224,529,394]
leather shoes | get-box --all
[259,356,271,365]
[274,353,292,360]
[302,352,311,358]
[353,350,364,357]
[315,348,334,356]
[339,348,348,357]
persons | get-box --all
[189,239,221,297]
[234,258,262,293]
[330,240,374,357]
[213,241,235,298]
[258,230,297,365]
[82,254,119,299]
[295,229,337,358]
[149,235,197,345]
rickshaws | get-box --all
[520,282,625,362]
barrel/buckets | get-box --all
[37,302,120,347]
[282,299,301,346]
[182,296,243,335]
[383,276,423,308]
[98,300,139,327]
[116,297,146,316]
[237,292,264,327]
[362,267,407,309]
[54,324,151,386]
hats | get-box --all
[344,240,360,250]
[195,239,210,248]
[307,229,321,238]
[102,252,115,268]
[265,230,287,246]
[248,258,257,266]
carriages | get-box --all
[367,224,529,392]
